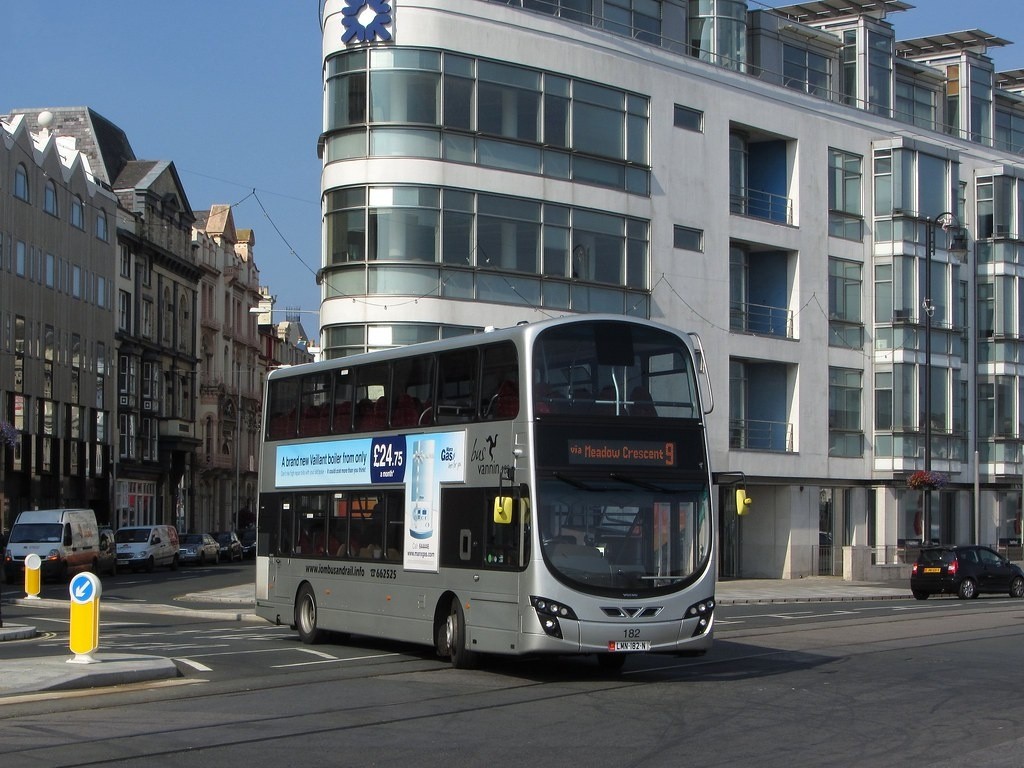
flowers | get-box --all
[906,470,951,489]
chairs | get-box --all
[289,524,340,558]
[268,379,657,440]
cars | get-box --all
[175,533,221,568]
[236,527,257,558]
[910,543,1024,600]
[208,531,245,563]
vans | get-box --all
[98,528,116,577]
[111,524,184,572]
[4,509,100,585]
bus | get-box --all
[254,314,752,673]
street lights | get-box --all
[922,211,972,544]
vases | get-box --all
[918,485,937,491]
